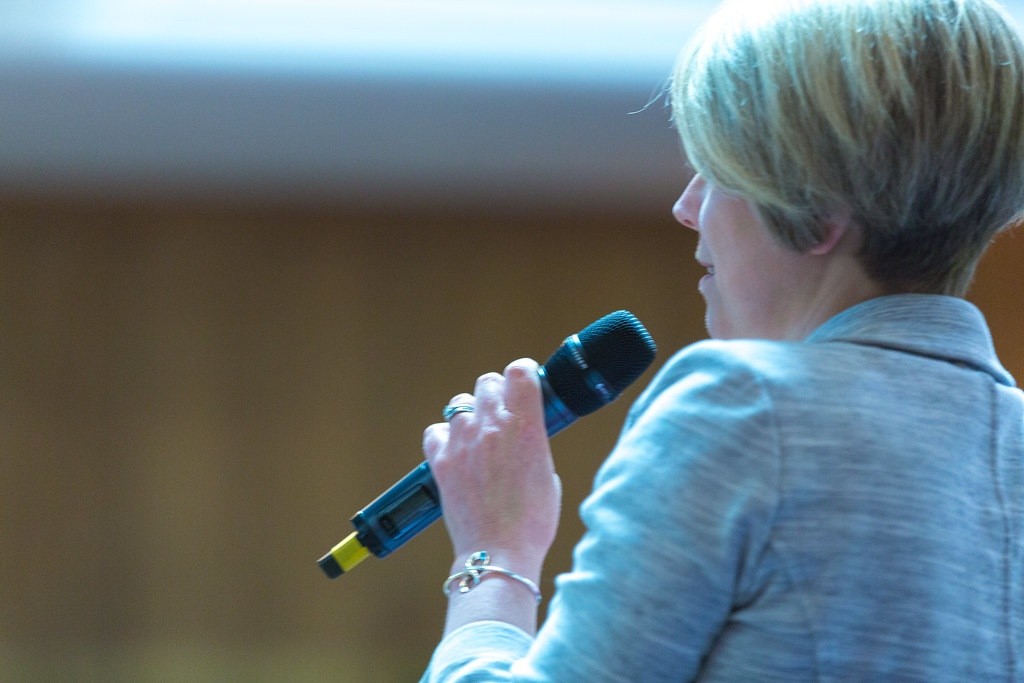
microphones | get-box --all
[314,309,658,578]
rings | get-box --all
[442,402,475,419]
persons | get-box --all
[418,1,1023,683]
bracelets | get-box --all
[442,551,542,602]
